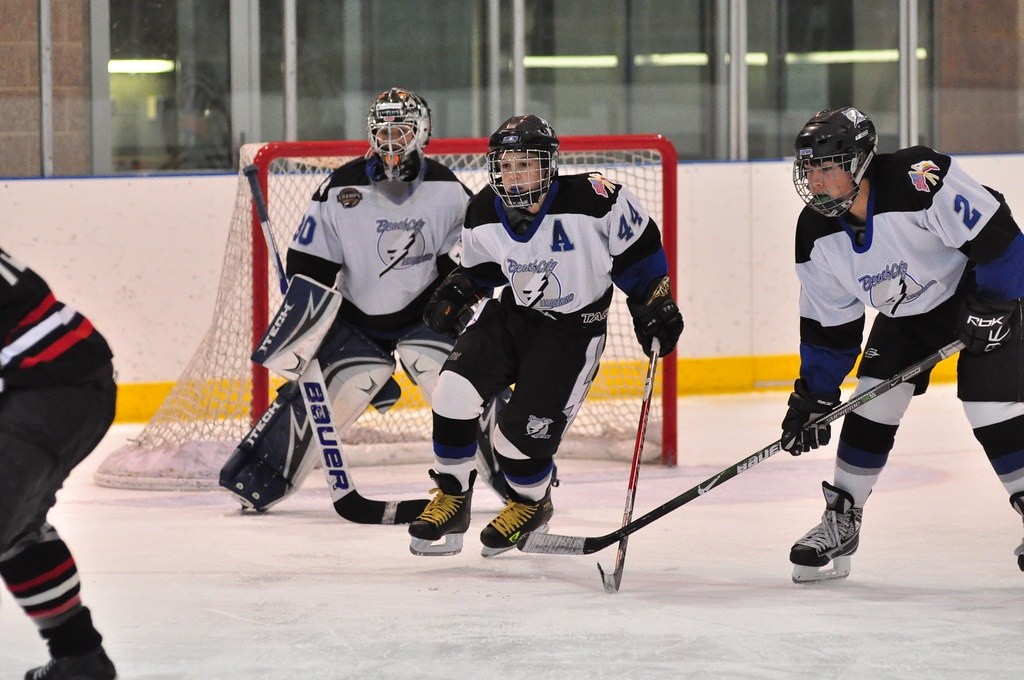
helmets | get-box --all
[486,116,560,208]
[793,106,877,217]
[365,87,432,166]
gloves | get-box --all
[782,379,841,457]
[625,275,683,358]
[422,265,481,334]
[959,293,1016,354]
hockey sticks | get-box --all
[595,334,659,597]
[339,346,515,605]
[243,162,435,526]
[516,338,962,556]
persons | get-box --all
[0,248,117,680]
[408,116,684,557]
[218,89,513,515]
[782,106,1024,583]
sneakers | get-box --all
[479,472,555,558]
[1008,490,1024,573]
[790,481,870,583]
[24,606,117,680]
[408,470,476,556]
